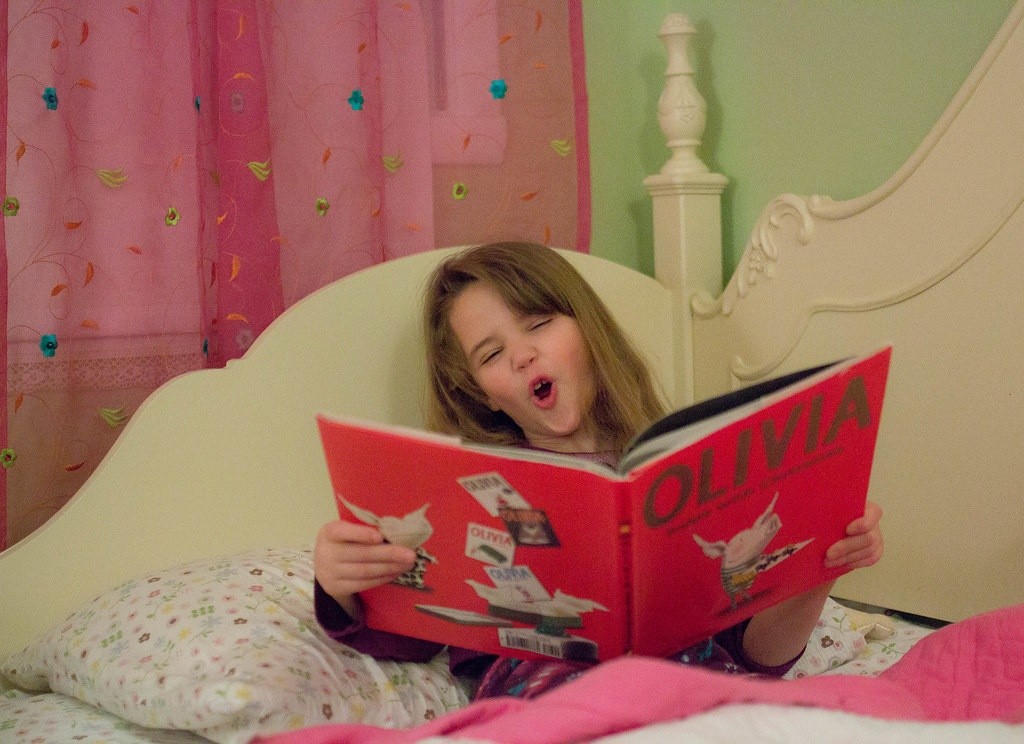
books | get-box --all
[315,336,903,669]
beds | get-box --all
[0,0,1024,744]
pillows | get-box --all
[0,548,893,744]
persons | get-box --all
[310,240,884,703]
